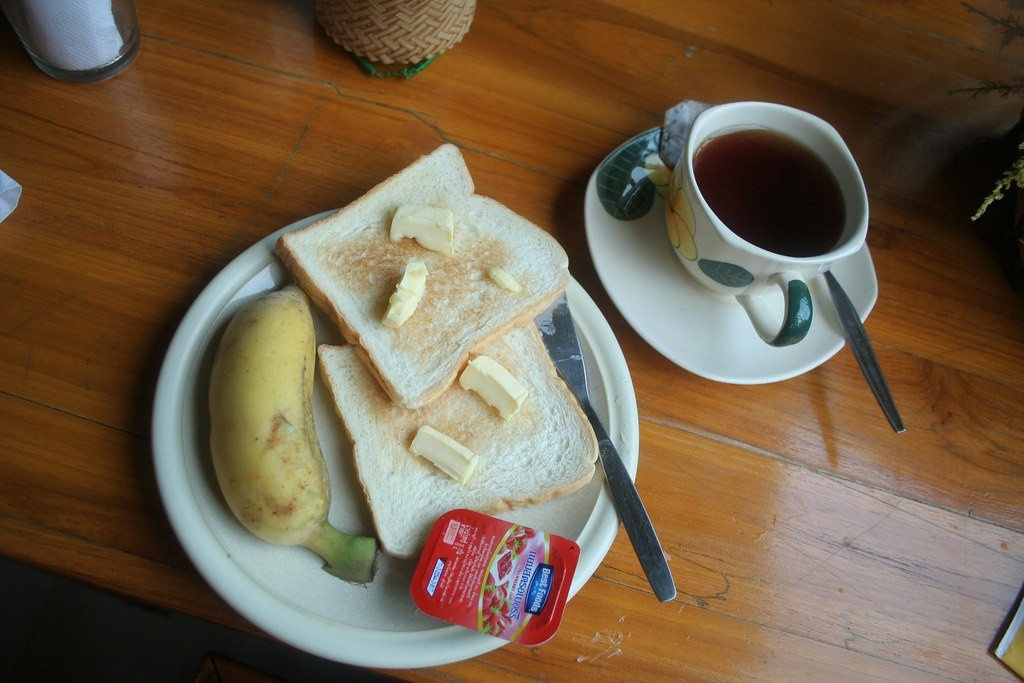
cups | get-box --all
[1,1,143,82]
[666,103,873,349]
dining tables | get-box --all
[1,0,1024,683]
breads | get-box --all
[274,143,599,560]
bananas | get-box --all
[209,286,378,583]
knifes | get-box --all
[536,293,676,602]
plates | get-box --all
[150,209,638,668]
[581,125,881,387]
